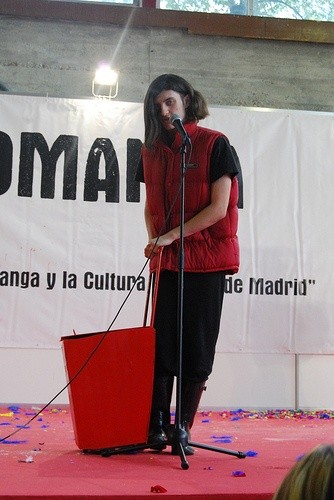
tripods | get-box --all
[101,141,246,470]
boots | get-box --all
[172,378,206,456]
[147,372,174,450]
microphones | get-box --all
[168,114,192,148]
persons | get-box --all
[143,73,244,454]
[271,444,334,499]
